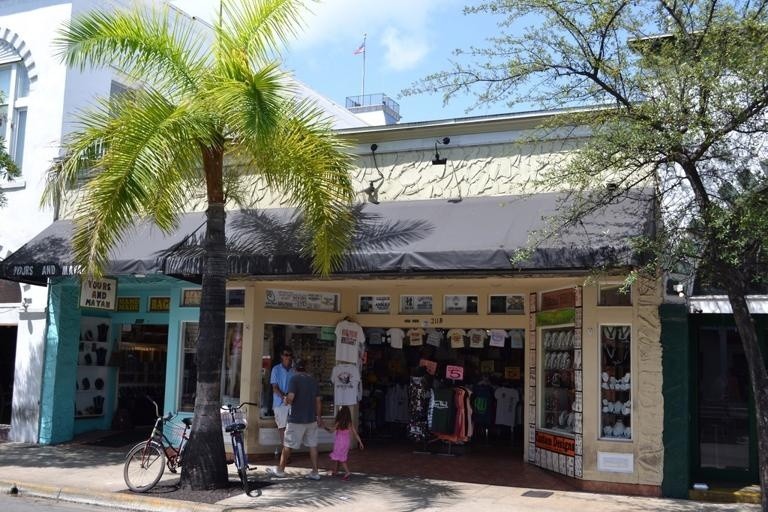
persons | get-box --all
[320,405,364,480]
[270,346,322,480]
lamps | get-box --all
[366,143,383,191]
[431,138,451,164]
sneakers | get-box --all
[272,468,286,478]
[305,472,320,481]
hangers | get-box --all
[345,314,355,323]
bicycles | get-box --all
[218,401,260,496]
[124,394,192,493]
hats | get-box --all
[296,359,304,368]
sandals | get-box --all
[341,471,352,480]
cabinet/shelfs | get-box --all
[120,342,166,399]
[540,324,633,441]
[74,314,113,418]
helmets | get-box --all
[283,354,292,356]
